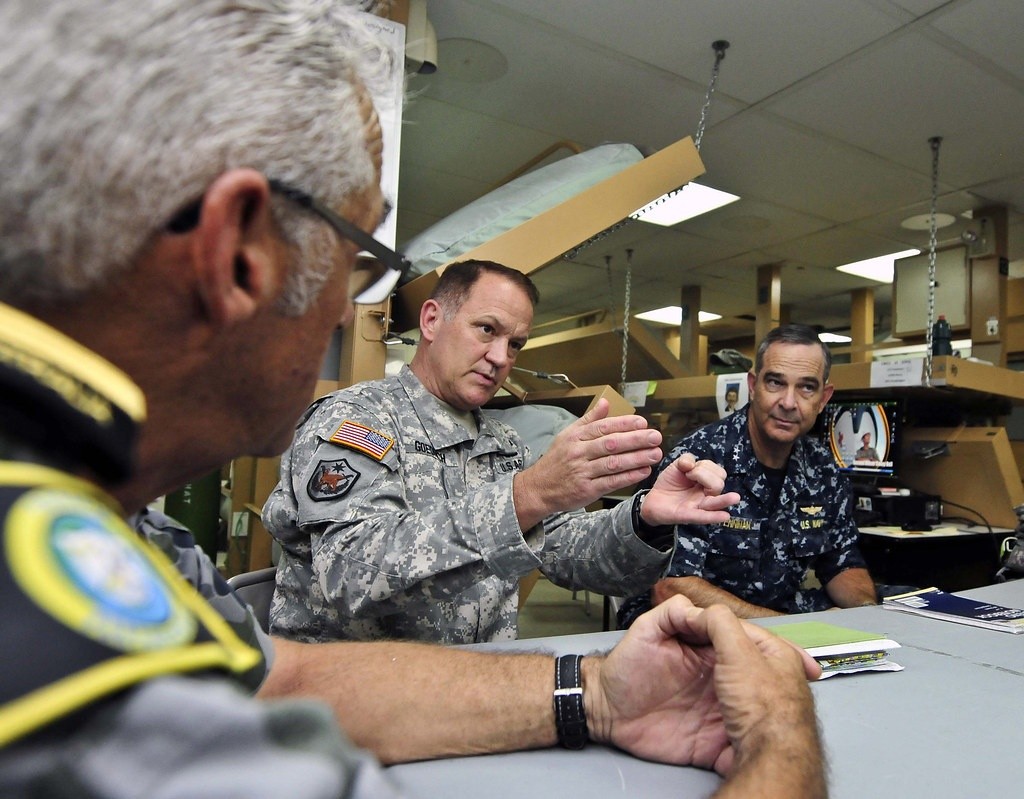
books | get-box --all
[882,586,1024,635]
[764,620,906,681]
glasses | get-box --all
[165,179,412,305]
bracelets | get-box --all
[553,653,590,751]
[632,489,663,536]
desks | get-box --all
[383,615,1024,798]
[805,578,1024,676]
[857,522,1016,606]
[597,495,634,631]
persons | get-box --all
[855,432,880,462]
[616,323,879,630]
[1,1,831,799]
[725,387,738,412]
[260,258,742,646]
